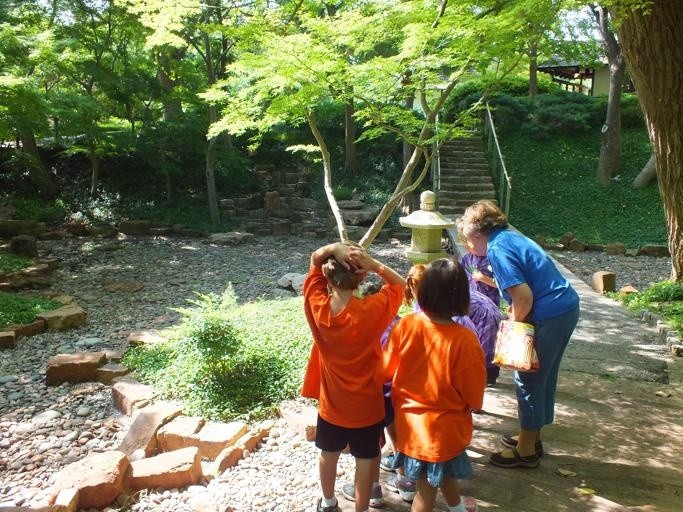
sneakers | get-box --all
[487,374,496,387]
[464,496,478,512]
[379,453,396,472]
[317,497,338,512]
[342,483,384,507]
[385,476,416,501]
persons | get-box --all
[304,240,406,512]
[342,218,508,508]
[463,198,579,468]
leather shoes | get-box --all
[488,447,540,468]
[501,433,543,455]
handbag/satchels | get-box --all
[491,319,540,373]
